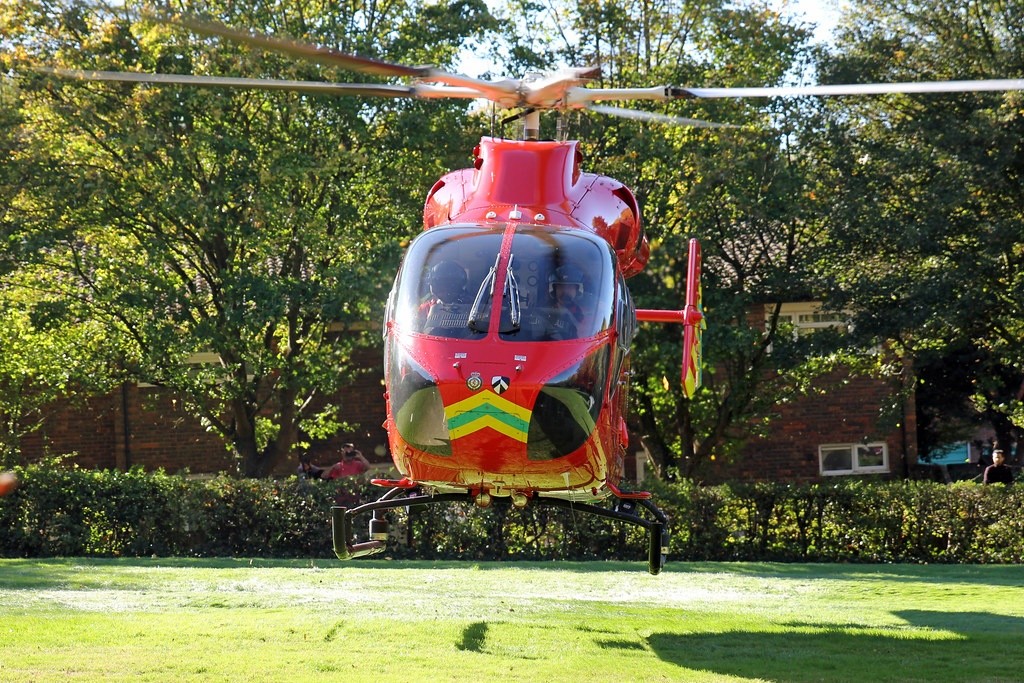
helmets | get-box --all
[548,265,585,300]
[428,261,468,296]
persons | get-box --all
[321,443,370,505]
[530,264,597,340]
[984,450,1014,485]
[414,258,472,337]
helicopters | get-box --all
[27,8,1024,575]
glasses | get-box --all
[435,282,456,288]
